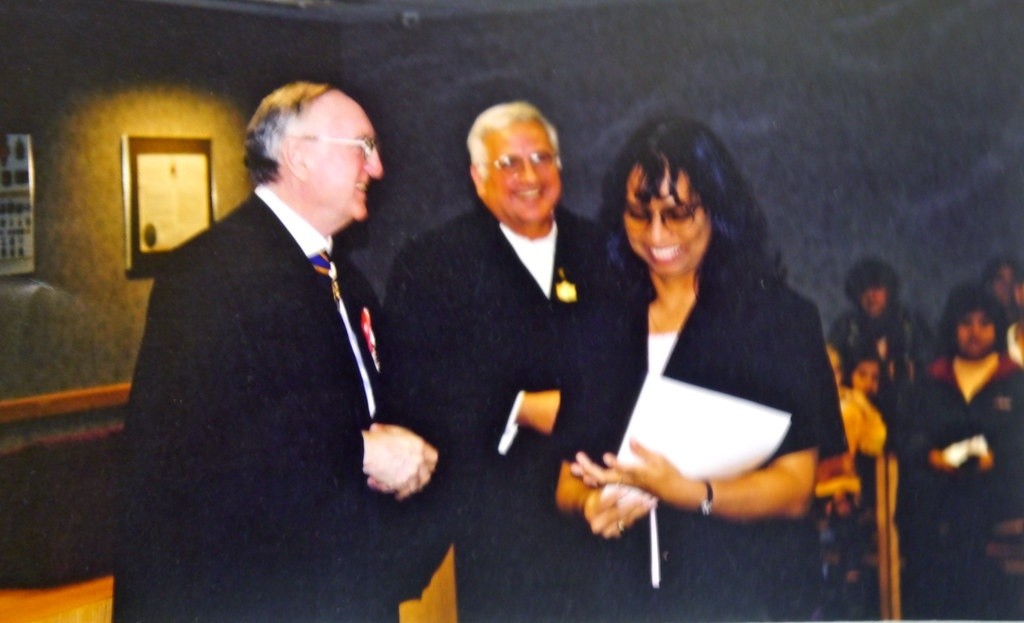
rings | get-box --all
[617,522,627,536]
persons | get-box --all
[828,250,1024,621]
[114,82,438,623]
[543,113,848,623]
[387,102,607,623]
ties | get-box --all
[306,249,342,313]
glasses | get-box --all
[484,150,560,173]
[280,133,379,154]
[624,199,701,231]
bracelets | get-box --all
[701,478,713,517]
